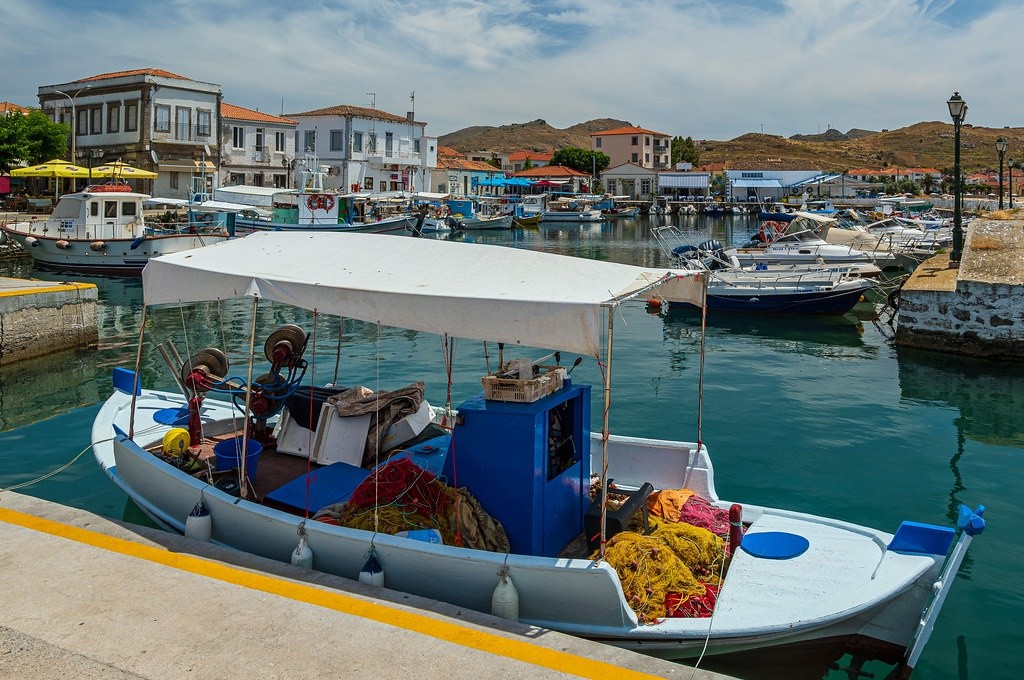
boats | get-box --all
[0,184,241,276]
[648,196,976,317]
[215,145,418,236]
[398,190,642,243]
[90,232,984,680]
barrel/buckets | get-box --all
[163,428,190,454]
[213,438,263,487]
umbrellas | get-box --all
[10,159,90,203]
[91,161,158,199]
[475,176,561,196]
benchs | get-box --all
[27,198,56,215]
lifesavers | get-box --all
[321,195,334,210]
[779,222,788,232]
[914,216,921,220]
[867,211,876,218]
[807,187,813,193]
[436,210,441,215]
[793,188,798,193]
[307,195,320,210]
[447,209,451,214]
[875,212,884,220]
[760,221,781,242]
[888,288,900,309]
[896,213,902,217]
[411,186,414,193]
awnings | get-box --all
[730,179,783,188]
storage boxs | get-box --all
[380,401,435,453]
[481,365,565,403]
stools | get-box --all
[585,478,657,552]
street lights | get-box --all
[994,137,1009,211]
[946,92,968,268]
[55,84,93,191]
[1007,158,1013,209]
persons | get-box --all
[143,210,180,235]
[19,186,30,196]
[42,185,55,203]
[621,202,627,208]
